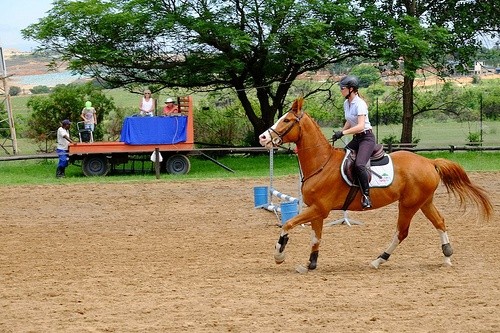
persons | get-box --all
[162,98,178,116]
[56,120,77,178]
[332,76,376,210]
[139,89,155,116]
[80,101,97,141]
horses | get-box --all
[260,98,494,271]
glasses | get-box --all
[144,93,150,94]
[340,87,347,91]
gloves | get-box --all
[332,131,344,142]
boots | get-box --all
[355,171,370,207]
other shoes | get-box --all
[56,172,61,177]
[61,173,65,178]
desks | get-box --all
[119,115,187,146]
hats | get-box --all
[85,101,92,110]
[61,120,72,125]
[164,98,175,104]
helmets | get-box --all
[339,76,359,87]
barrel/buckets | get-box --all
[254,186,268,207]
[281,202,299,224]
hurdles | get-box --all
[263,145,306,227]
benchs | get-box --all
[382,143,417,151]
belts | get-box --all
[353,130,372,138]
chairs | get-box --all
[76,120,94,144]
